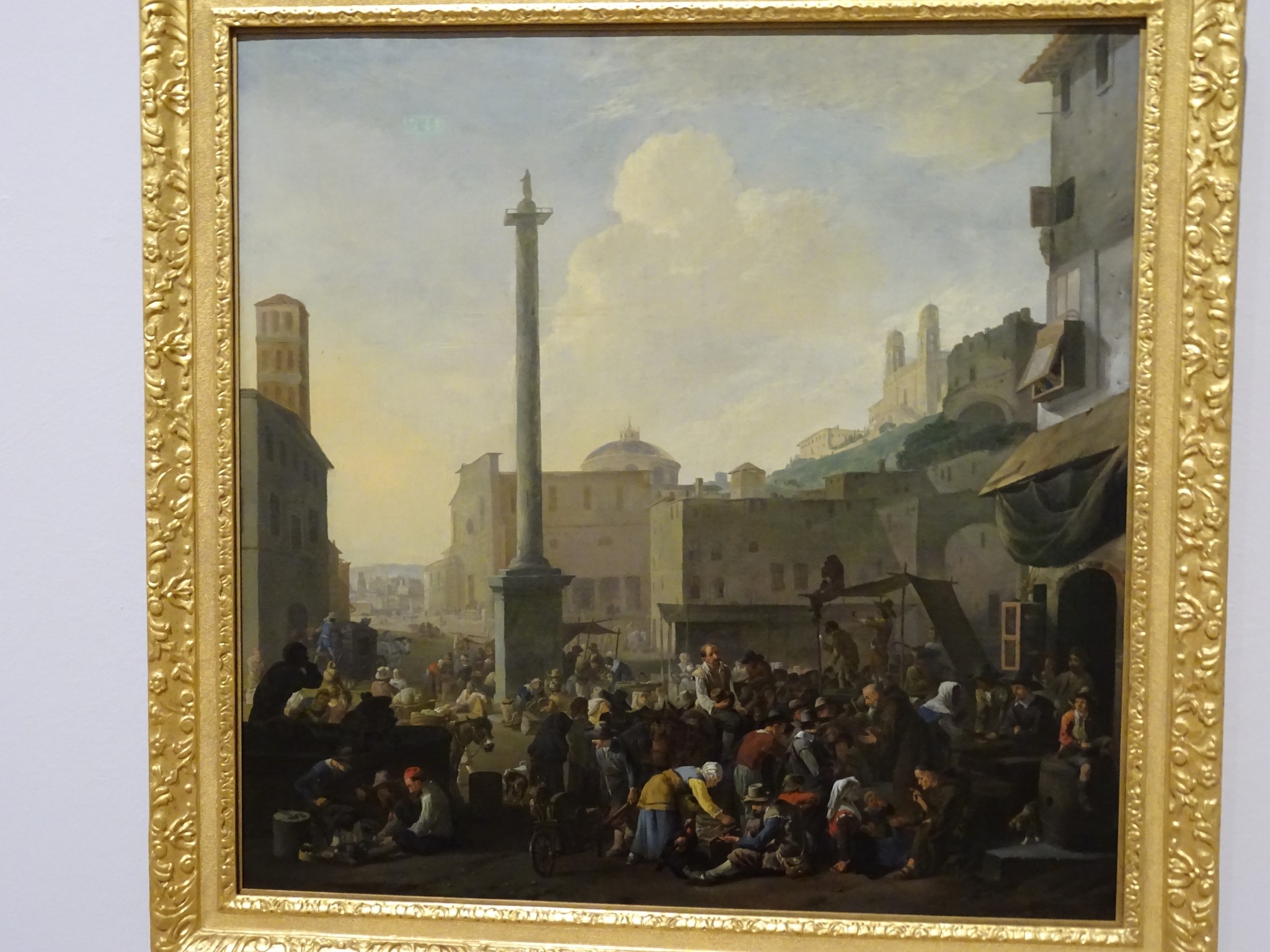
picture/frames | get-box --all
[138,0,1252,952]
